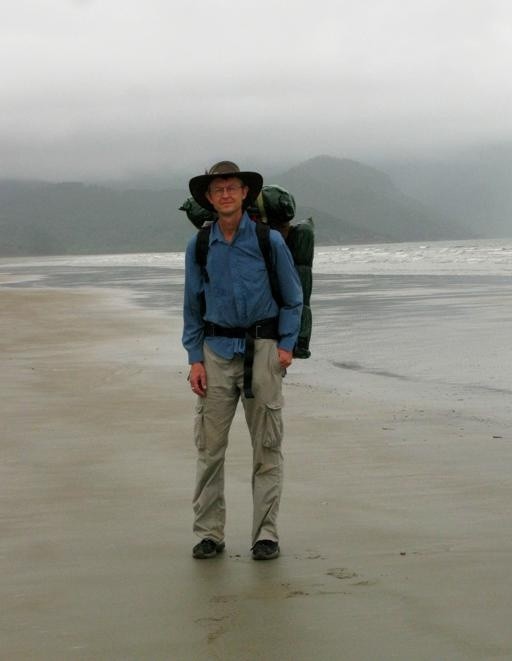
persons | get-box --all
[182,161,304,559]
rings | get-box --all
[192,386,194,390]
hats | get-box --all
[186,158,265,217]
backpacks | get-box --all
[178,182,317,401]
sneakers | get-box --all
[191,538,226,559]
[249,538,280,562]
